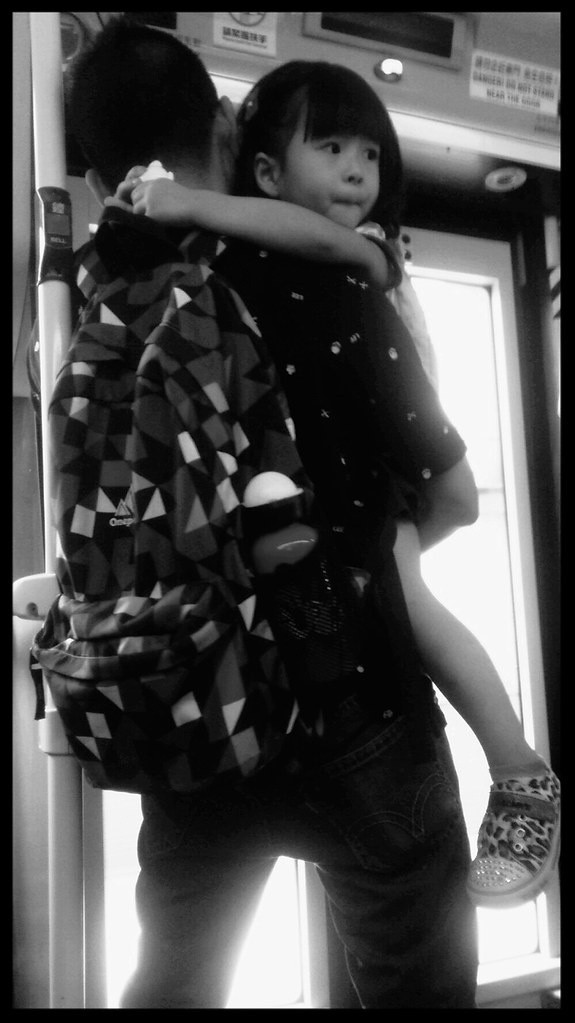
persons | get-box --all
[64,14,561,1009]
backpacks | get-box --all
[25,226,355,793]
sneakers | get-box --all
[466,754,561,909]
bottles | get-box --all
[240,471,355,683]
